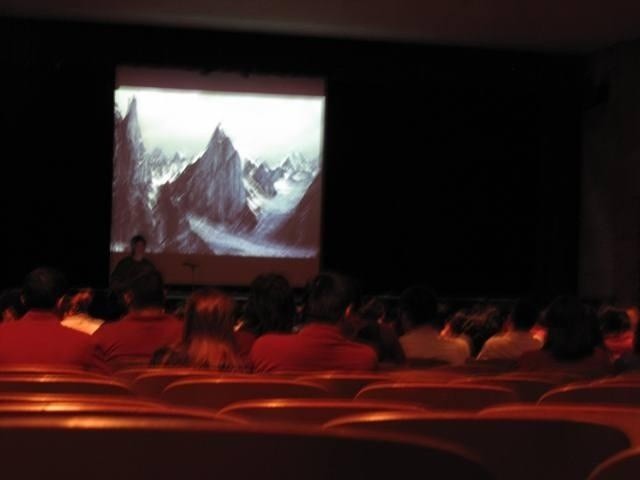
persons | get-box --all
[2,261,639,379]
[112,233,158,288]
